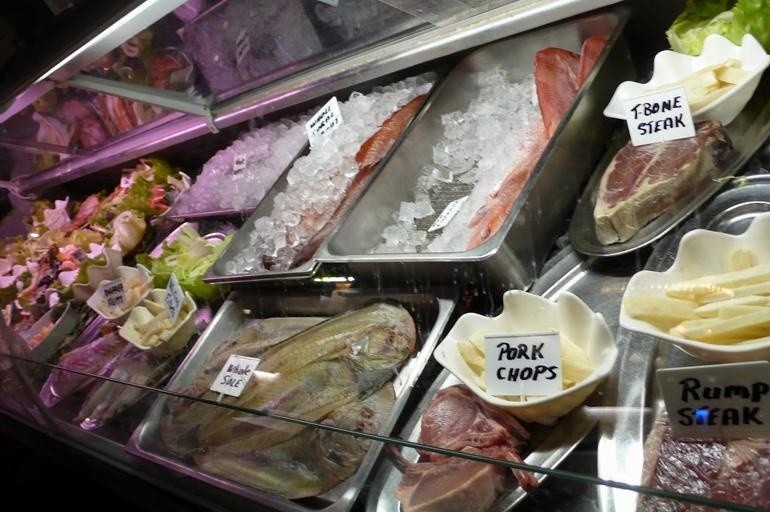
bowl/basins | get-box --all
[0,195,198,355]
[597,33,768,127]
[436,286,620,423]
[618,210,768,360]
